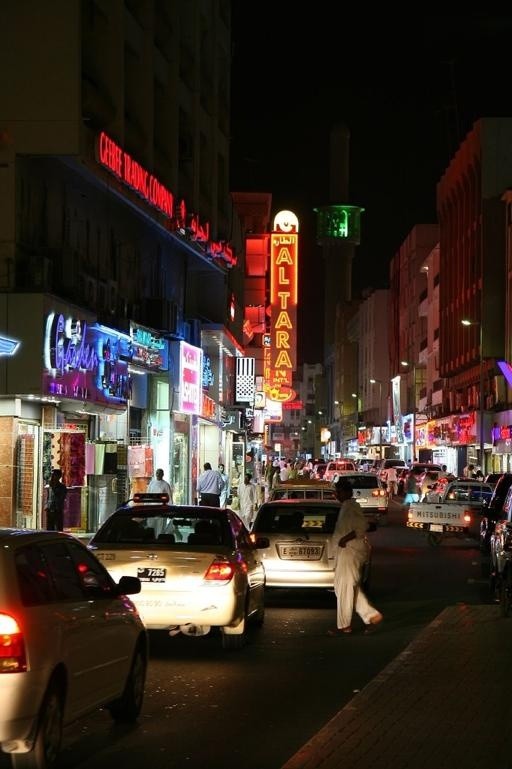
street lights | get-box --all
[400,359,417,461]
[370,377,384,458]
[293,389,364,461]
[461,319,486,477]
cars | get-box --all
[353,457,451,498]
[272,447,390,515]
[250,492,368,608]
[84,500,273,646]
[482,471,512,617]
[0,527,151,761]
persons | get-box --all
[144,469,174,539]
[326,478,384,638]
[197,447,486,532]
[43,469,68,532]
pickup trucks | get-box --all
[409,478,496,546]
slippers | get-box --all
[363,623,380,635]
[327,630,352,637]
